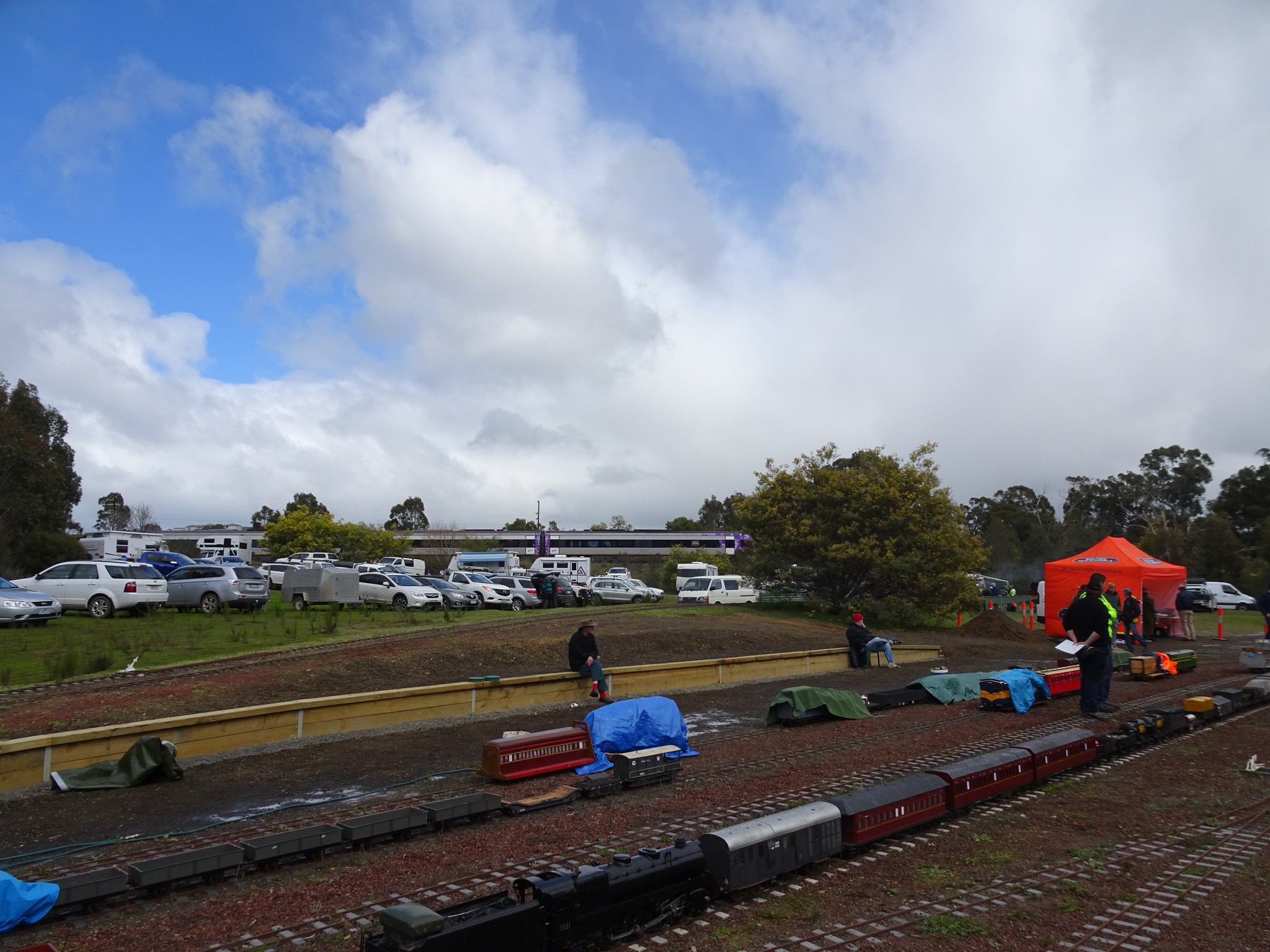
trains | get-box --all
[360,678,1270,951]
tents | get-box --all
[1045,536,1187,638]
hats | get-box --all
[1178,585,1186,588]
[1142,588,1150,593]
[854,613,863,622]
[1087,580,1101,591]
[1108,583,1115,589]
[577,619,598,630]
[1121,588,1132,593]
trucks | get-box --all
[676,562,718,593]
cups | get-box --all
[571,702,578,708]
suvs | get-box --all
[159,564,269,615]
[8,559,169,620]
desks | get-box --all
[1155,616,1181,637]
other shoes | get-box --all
[1097,703,1114,712]
[1081,711,1111,720]
[590,690,599,698]
[1111,646,1123,651]
[888,661,898,668]
[599,696,614,703]
[1143,646,1148,651]
[1127,649,1133,652]
[1104,700,1120,710]
[888,640,894,645]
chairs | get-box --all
[857,643,880,668]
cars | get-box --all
[138,551,249,578]
[259,552,665,612]
[0,577,62,629]
[1185,587,1219,613]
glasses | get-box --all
[1111,587,1115,589]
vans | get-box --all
[676,575,760,606]
[1186,582,1257,611]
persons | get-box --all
[1062,573,1117,721]
[976,582,1016,612]
[540,578,559,609]
[1175,584,1196,641]
[1123,588,1148,654]
[1257,589,1270,640]
[1141,587,1156,642]
[845,613,900,669]
[568,618,614,704]
[1102,583,1123,649]
